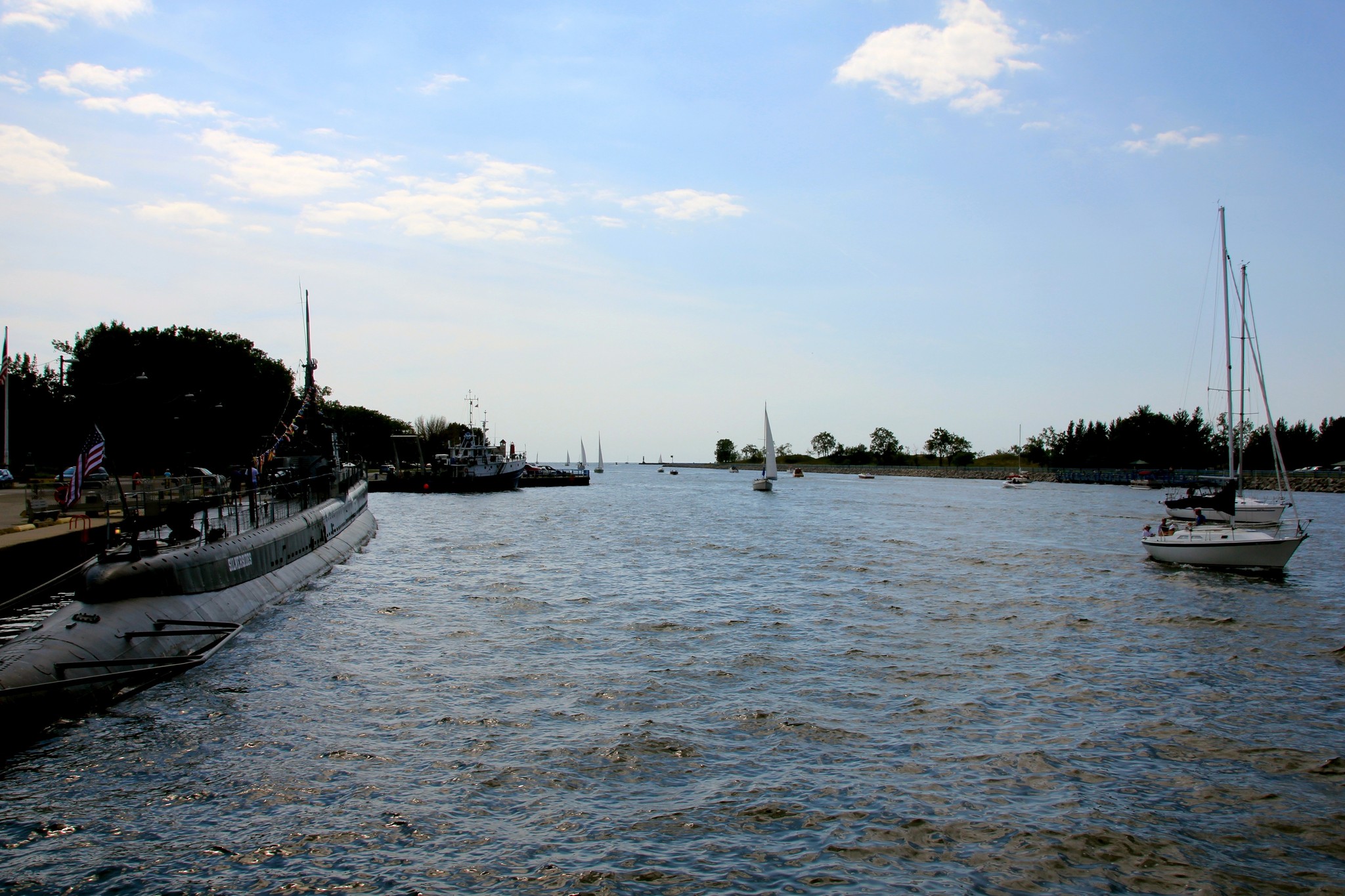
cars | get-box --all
[171,467,226,487]
[54,466,110,489]
[379,465,396,474]
[0,469,14,489]
[1292,466,1345,473]
[523,462,556,477]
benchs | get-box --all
[27,499,61,523]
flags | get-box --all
[66,423,106,507]
[253,385,315,474]
[0,337,7,384]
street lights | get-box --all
[59,355,80,483]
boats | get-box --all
[858,473,875,479]
[1129,470,1164,490]
[792,468,804,477]
[431,389,527,492]
[657,467,664,473]
[0,287,380,726]
[729,465,739,473]
[1003,475,1027,489]
[670,469,678,475]
[786,466,794,473]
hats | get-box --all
[1169,525,1175,529]
[1162,518,1167,521]
[1194,509,1201,512]
[1143,524,1152,530]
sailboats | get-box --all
[536,451,539,464]
[565,449,570,466]
[1011,424,1035,484]
[575,436,587,469]
[753,399,778,491]
[1157,261,1294,527]
[625,455,629,464]
[1140,206,1315,572]
[594,430,604,473]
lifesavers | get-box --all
[54,486,69,505]
[464,468,468,473]
[133,472,141,485]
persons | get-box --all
[230,465,242,505]
[1158,517,1169,536]
[245,463,259,505]
[1142,524,1156,537]
[1184,521,1193,529]
[163,469,171,489]
[1194,509,1206,525]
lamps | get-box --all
[114,527,121,534]
